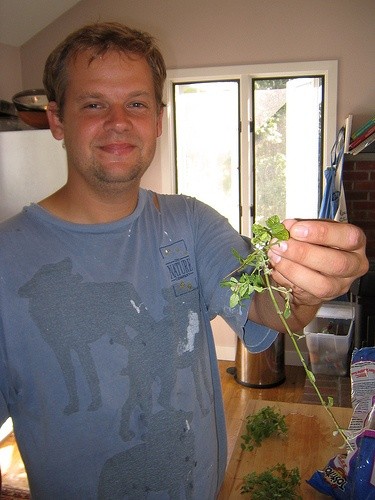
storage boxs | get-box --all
[304,302,356,375]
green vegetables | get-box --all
[240,405,289,452]
[219,215,353,451]
[240,461,304,500]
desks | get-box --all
[215,398,354,500]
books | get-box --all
[349,118,375,155]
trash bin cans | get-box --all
[226,324,285,389]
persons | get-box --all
[1,21,370,499]
